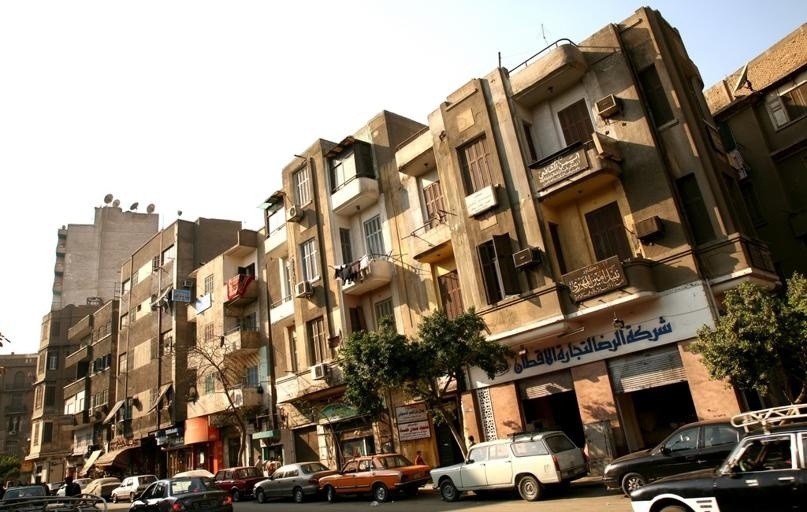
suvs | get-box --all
[625,401,806,512]
[427,429,588,502]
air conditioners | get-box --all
[510,247,543,271]
[293,280,314,299]
[285,206,303,224]
[592,89,619,121]
[311,363,332,380]
[632,214,666,240]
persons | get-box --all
[63,474,81,502]
[413,449,427,466]
[467,435,477,451]
[254,453,267,475]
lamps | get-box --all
[517,344,527,357]
[612,310,625,329]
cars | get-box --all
[252,461,334,502]
[0,474,158,511]
[315,453,432,502]
[598,413,757,496]
[204,465,267,502]
[127,476,235,511]
